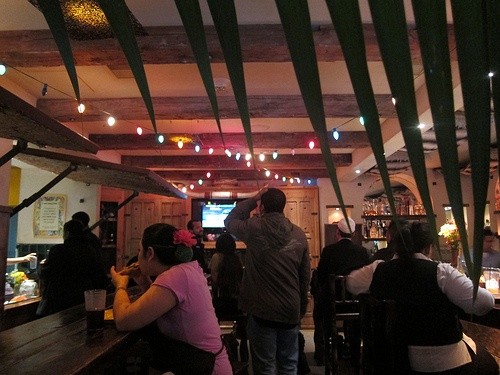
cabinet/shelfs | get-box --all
[361,215,438,242]
[96,184,125,278]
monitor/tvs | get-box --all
[200,203,237,232]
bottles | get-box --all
[101,234,108,245]
[108,233,114,245]
[361,197,427,255]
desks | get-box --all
[3,295,42,321]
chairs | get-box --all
[218,320,250,363]
[220,328,251,375]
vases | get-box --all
[451,247,462,268]
[14,283,21,296]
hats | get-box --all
[338,217,356,234]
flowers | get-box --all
[172,230,198,249]
[6,269,29,285]
[438,220,462,248]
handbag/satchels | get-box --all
[147,329,215,375]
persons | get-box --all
[225,188,309,375]
[72,212,108,296]
[347,220,500,375]
[211,235,249,364]
[7,252,36,265]
[36,221,95,319]
[186,221,212,274]
[109,223,234,375]
[310,219,371,368]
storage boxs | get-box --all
[325,205,353,225]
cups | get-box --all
[481,266,500,299]
[462,248,473,275]
[30,257,37,269]
[85,289,106,331]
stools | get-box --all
[313,274,397,375]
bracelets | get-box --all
[115,287,128,296]
